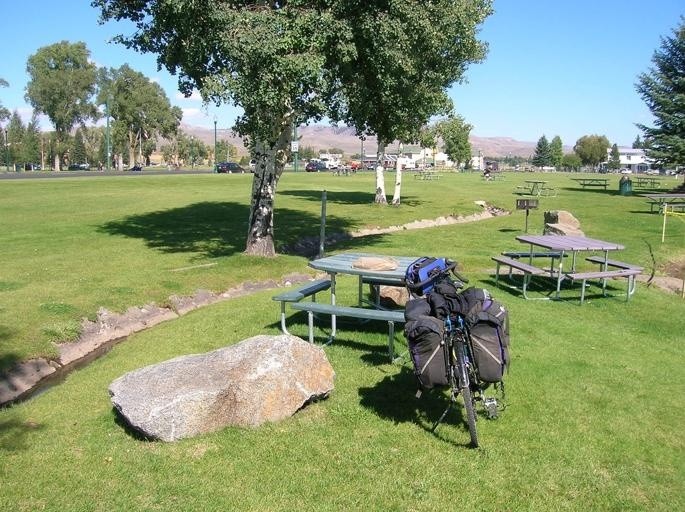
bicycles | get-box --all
[404,257,499,449]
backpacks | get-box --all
[402,286,512,391]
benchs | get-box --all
[491,250,644,302]
[272,278,407,364]
[571,178,610,192]
[632,178,661,187]
[413,170,443,180]
[645,201,685,214]
[517,185,559,197]
[480,173,507,182]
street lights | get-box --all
[106,94,115,168]
[213,114,217,170]
[191,136,194,168]
[4,127,9,171]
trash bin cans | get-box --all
[619,175,632,196]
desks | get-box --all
[524,181,548,195]
[515,235,624,290]
[657,194,685,213]
[309,253,421,325]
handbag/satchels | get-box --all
[403,253,449,296]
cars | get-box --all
[366,164,375,169]
[26,162,41,170]
[218,162,245,173]
[249,160,255,173]
[70,162,89,170]
[622,168,632,174]
[306,160,328,171]
